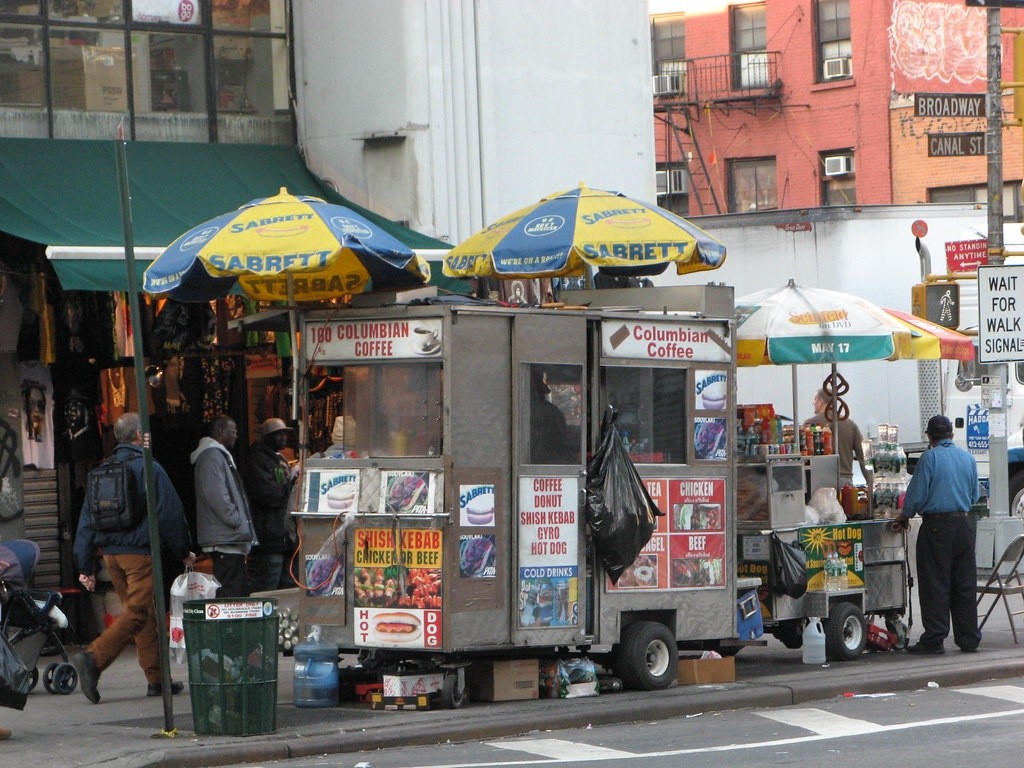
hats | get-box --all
[261,418,294,440]
[925,415,953,434]
[333,416,356,446]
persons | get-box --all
[247,418,299,592]
[190,416,259,598]
[896,415,983,654]
[74,414,196,702]
[309,415,357,458]
[531,373,567,464]
[804,389,869,488]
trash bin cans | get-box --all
[181,598,280,735]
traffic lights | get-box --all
[911,283,960,332]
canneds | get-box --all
[539,581,569,622]
[751,443,798,463]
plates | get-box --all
[411,340,441,354]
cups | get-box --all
[412,327,439,349]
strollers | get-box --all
[0,539,79,694]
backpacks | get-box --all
[88,455,159,535]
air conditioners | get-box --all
[653,75,679,95]
[824,58,850,78]
[825,156,853,176]
[656,170,682,196]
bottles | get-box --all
[833,552,842,590]
[802,617,826,665]
[841,424,909,520]
[734,403,832,463]
[521,580,568,626]
[841,559,848,590]
[294,625,339,707]
[822,553,837,591]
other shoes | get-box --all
[907,640,946,654]
[960,646,974,652]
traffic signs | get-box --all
[945,239,989,273]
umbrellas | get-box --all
[441,181,727,286]
[832,308,975,500]
[733,279,913,447]
[144,188,431,423]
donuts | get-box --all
[619,556,657,586]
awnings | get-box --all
[0,137,474,295]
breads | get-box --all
[327,491,355,509]
[466,503,493,524]
[702,388,725,411]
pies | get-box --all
[697,423,724,456]
[390,476,425,509]
[309,559,342,592]
[463,539,493,574]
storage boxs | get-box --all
[0,42,136,113]
[737,588,763,642]
[673,654,735,685]
[471,659,539,701]
[383,670,443,697]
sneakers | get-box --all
[73,652,101,704]
[147,681,184,697]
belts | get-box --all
[923,512,968,521]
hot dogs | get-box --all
[374,612,422,642]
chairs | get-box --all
[972,533,1024,643]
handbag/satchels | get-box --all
[170,562,222,663]
[585,409,665,585]
[772,532,809,600]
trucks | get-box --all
[637,199,1023,521]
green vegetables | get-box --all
[354,565,409,592]
